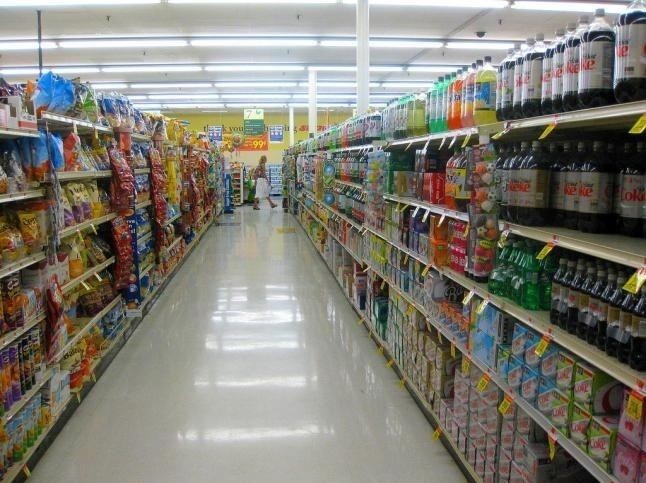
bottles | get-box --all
[313,0,646,372]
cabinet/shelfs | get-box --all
[382,101,645,483]
[218,161,244,208]
[282,106,392,338]
[0,110,245,483]
[1,110,128,482]
[119,111,221,316]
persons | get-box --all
[251,155,278,211]
